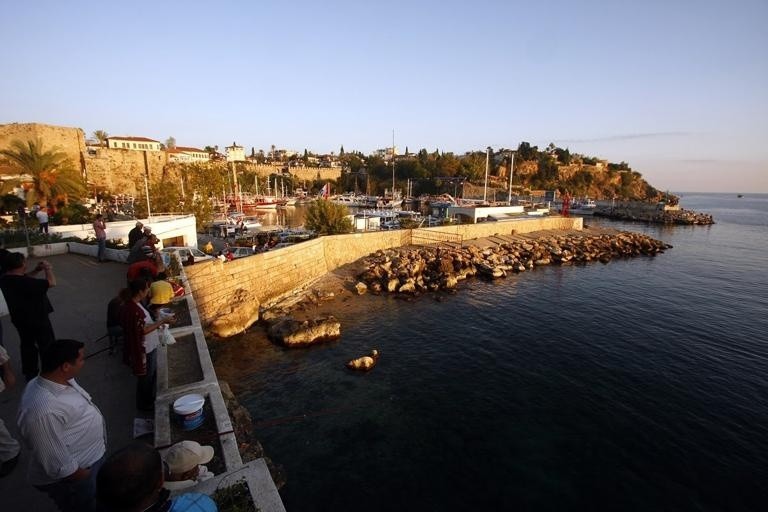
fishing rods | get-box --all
[156,402,386,450]
[81,259,240,359]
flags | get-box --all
[318,184,329,198]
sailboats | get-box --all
[193,176,319,209]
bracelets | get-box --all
[35,266,42,271]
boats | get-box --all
[548,198,596,216]
[328,193,403,207]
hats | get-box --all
[162,439,214,474]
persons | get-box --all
[92,213,111,262]
[0,345,18,399]
[35,205,50,235]
[0,418,23,482]
[2,250,58,382]
[187,253,194,265]
[225,249,233,260]
[91,439,175,511]
[105,222,178,409]
[16,339,111,511]
[204,241,214,254]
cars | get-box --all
[216,246,253,258]
[212,212,316,247]
[160,247,215,263]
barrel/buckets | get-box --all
[173,393,205,430]
